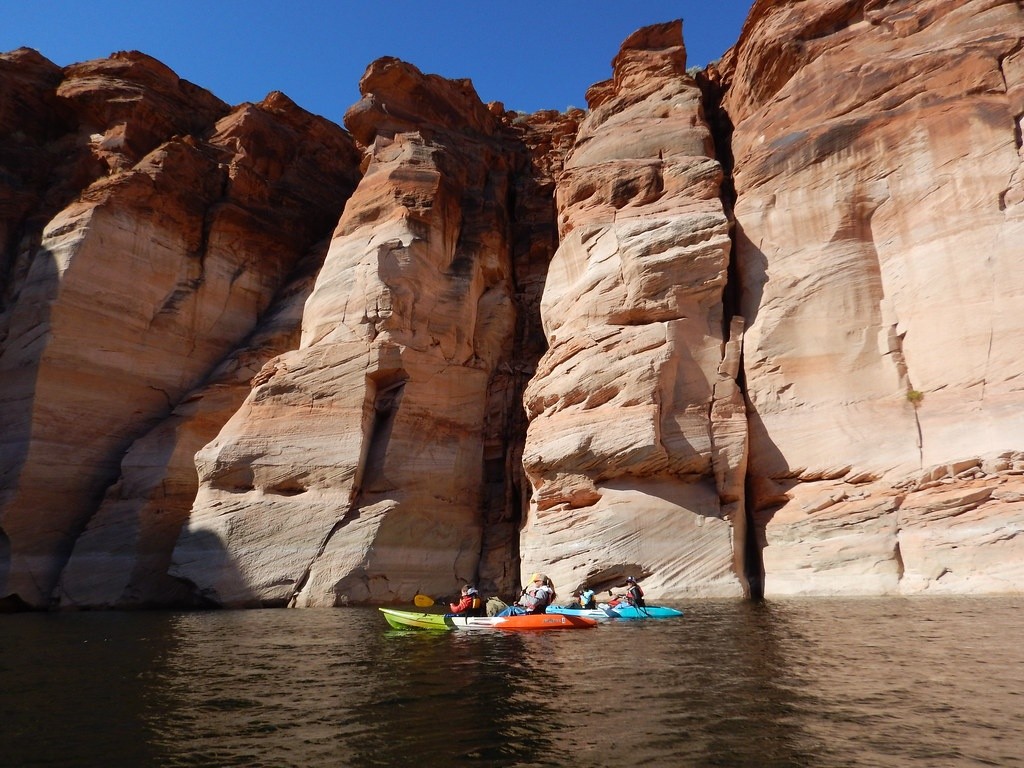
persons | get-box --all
[598,576,644,609]
[514,573,555,612]
[450,584,480,615]
[565,587,596,610]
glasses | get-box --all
[627,581,633,583]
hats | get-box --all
[626,576,636,583]
[533,573,547,582]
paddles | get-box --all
[514,573,538,603]
[414,594,451,607]
[608,591,621,598]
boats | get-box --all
[491,604,685,620]
[376,605,598,631]
